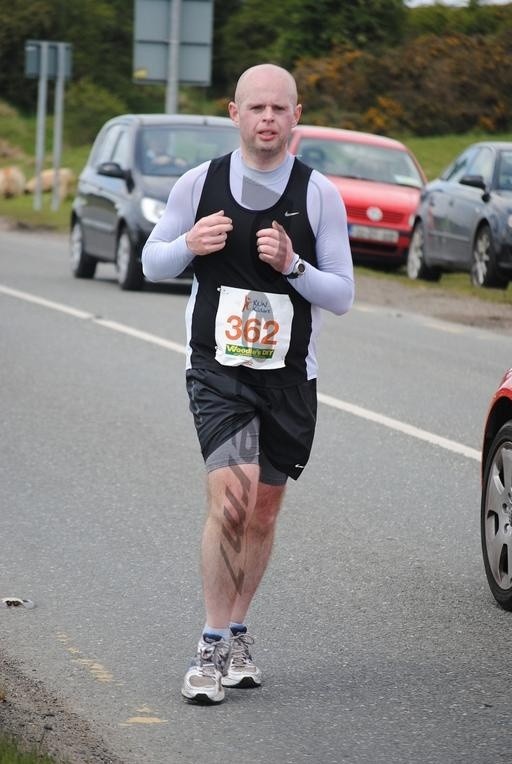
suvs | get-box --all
[68,113,241,288]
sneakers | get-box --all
[181,633,229,704]
[220,624,262,687]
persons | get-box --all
[140,56,356,704]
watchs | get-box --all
[284,257,306,280]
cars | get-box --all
[283,126,428,273]
[478,372,511,607]
[403,141,512,286]
